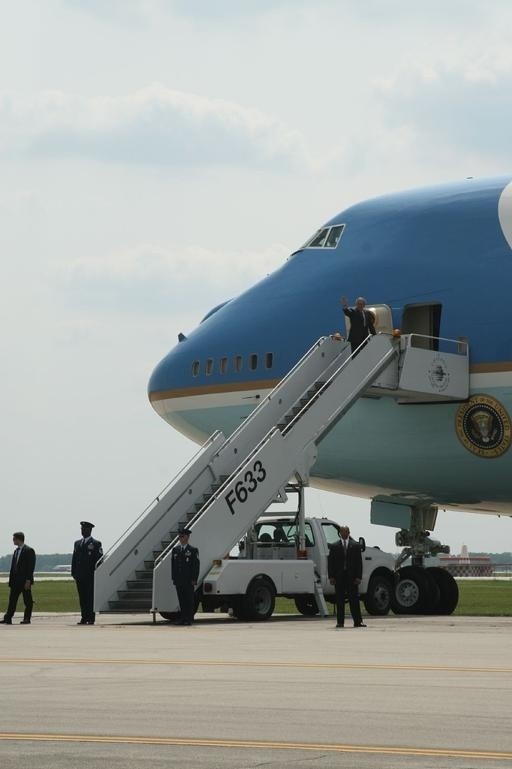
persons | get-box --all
[71,521,103,624]
[1,532,36,624]
[172,528,200,624]
[327,525,366,627]
[340,295,376,359]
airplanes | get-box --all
[93,177,512,620]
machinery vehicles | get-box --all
[204,514,398,620]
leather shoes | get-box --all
[77,619,95,625]
[336,624,344,627]
[20,620,31,624]
[354,622,367,627]
[0,619,12,624]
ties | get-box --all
[181,547,184,552]
[15,548,20,563]
[344,541,347,570]
[81,539,85,552]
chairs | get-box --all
[260,528,310,543]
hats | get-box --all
[176,528,192,534]
[80,521,95,527]
